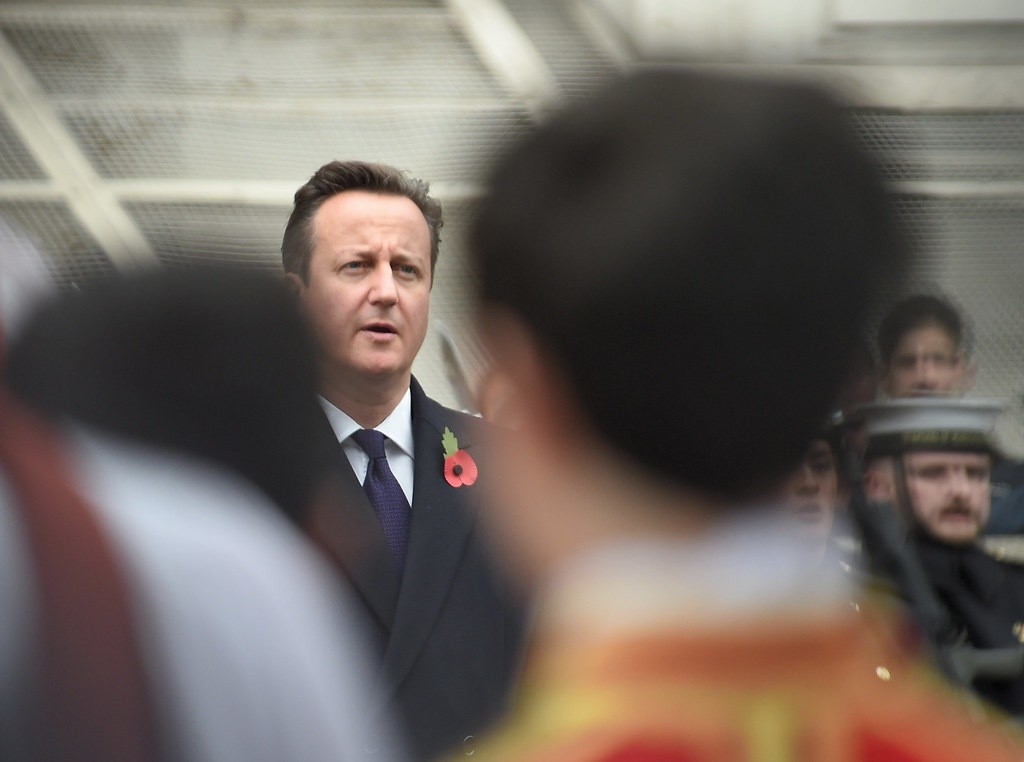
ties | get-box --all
[350,426,413,574]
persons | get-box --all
[1,163,1023,761]
[442,61,1023,760]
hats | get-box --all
[858,394,1005,460]
